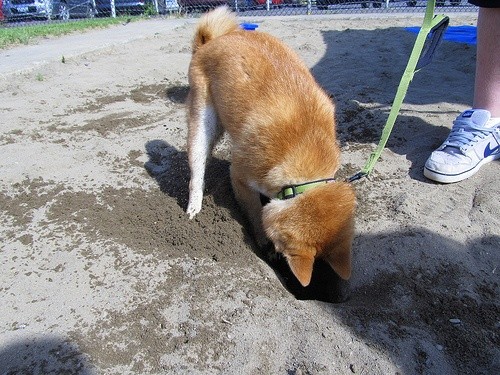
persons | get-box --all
[423,0,500,183]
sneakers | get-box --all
[422,108,500,184]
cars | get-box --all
[1,0,96,22]
[94,0,167,19]
[163,1,479,15]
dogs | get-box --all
[185,3,357,287]
